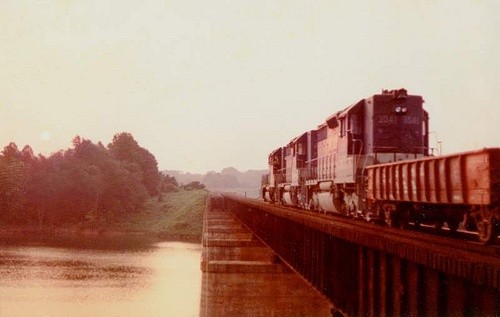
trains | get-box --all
[261,89,497,245]
